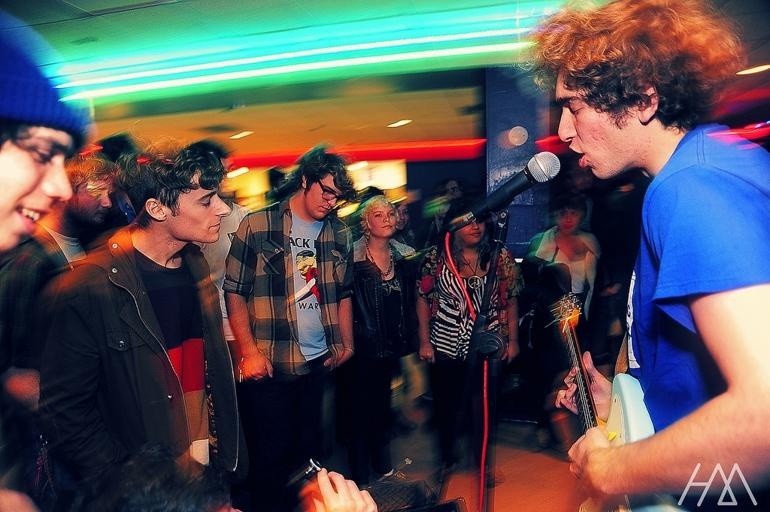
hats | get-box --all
[0,11,91,153]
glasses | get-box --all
[318,179,344,201]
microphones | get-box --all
[449,148,562,234]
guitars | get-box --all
[550,297,685,512]
